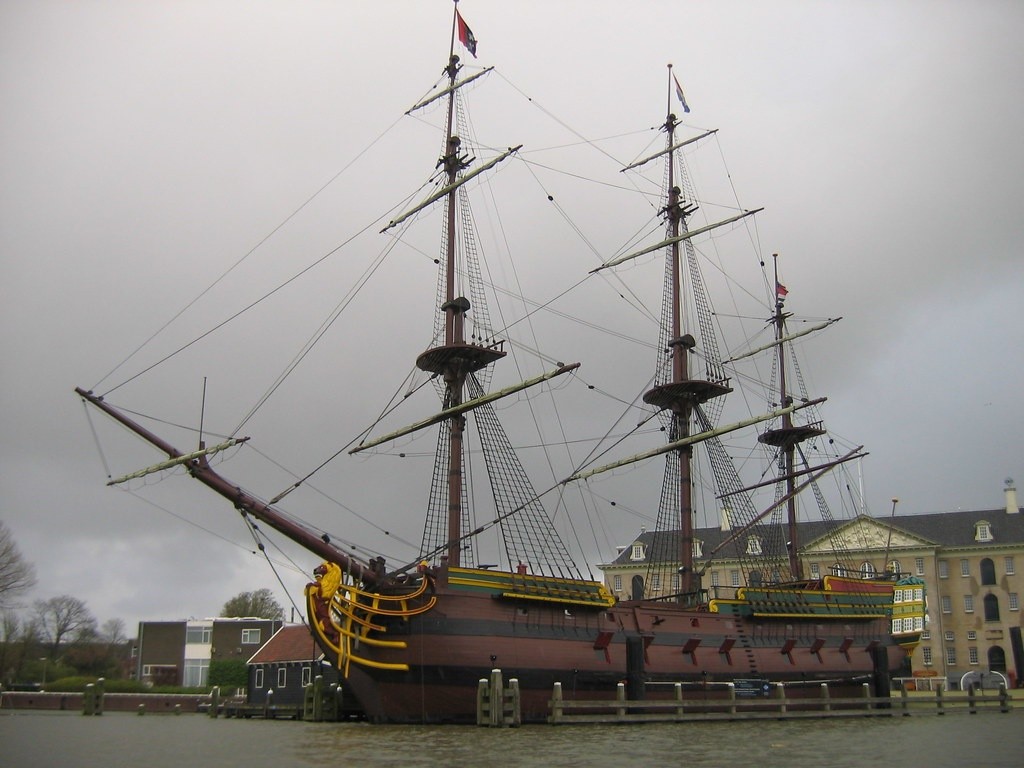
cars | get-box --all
[7,677,41,691]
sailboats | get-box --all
[75,0,928,717]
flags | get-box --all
[457,11,478,58]
[673,74,690,112]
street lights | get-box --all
[39,658,47,691]
